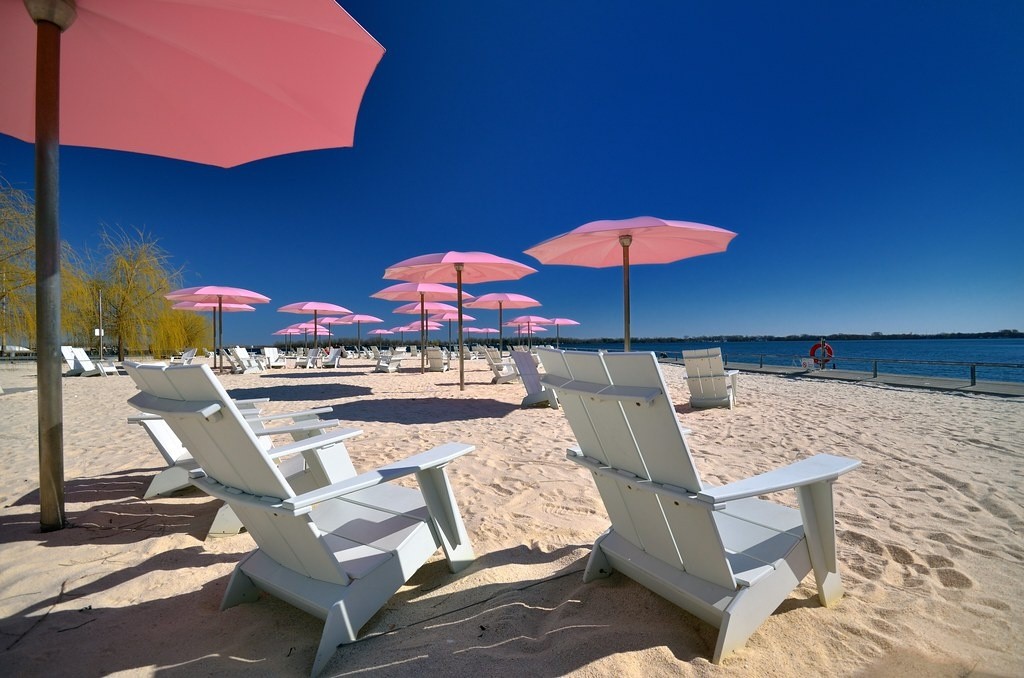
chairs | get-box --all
[62,345,862,678]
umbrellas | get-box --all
[381,250,539,392]
[164,284,271,373]
[0,0,387,534]
[273,300,385,359]
[522,215,738,352]
[368,283,583,374]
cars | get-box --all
[89,346,132,356]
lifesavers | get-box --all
[810,343,832,363]
